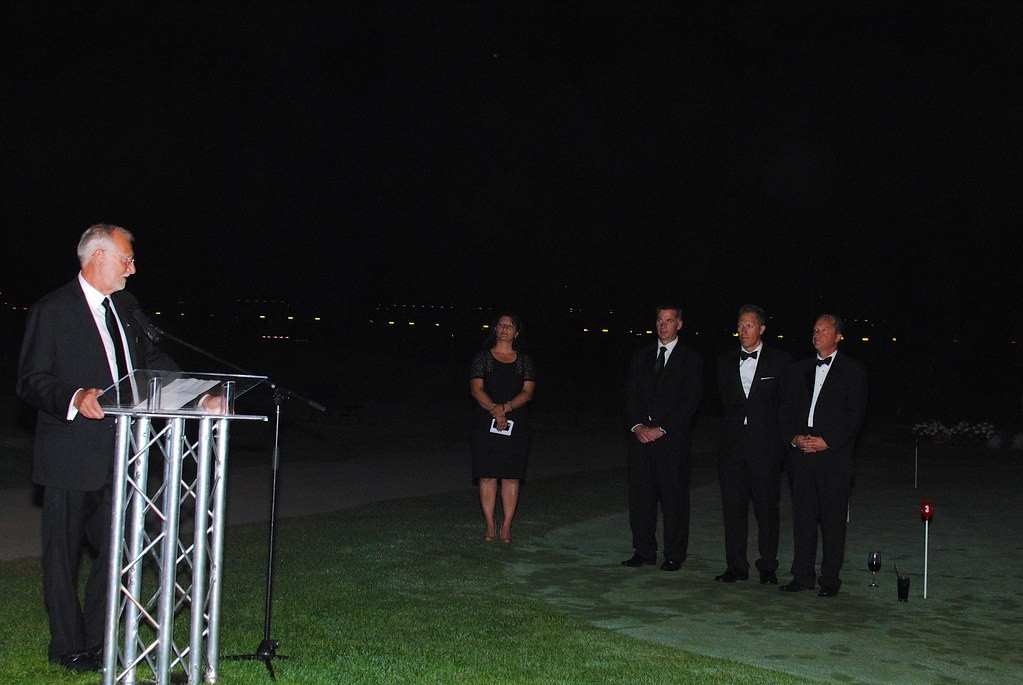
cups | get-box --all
[897,576,910,603]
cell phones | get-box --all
[493,420,511,431]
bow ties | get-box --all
[739,350,757,360]
[816,357,832,367]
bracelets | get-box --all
[502,402,505,411]
[507,401,512,411]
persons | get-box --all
[715,305,795,583]
[620,297,702,572]
[468,311,536,545]
[16,223,230,673]
[778,313,869,595]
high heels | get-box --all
[500,523,511,544]
[484,519,496,543]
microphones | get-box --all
[129,304,162,344]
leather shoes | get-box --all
[621,551,656,567]
[779,580,815,592]
[714,574,749,582]
[818,588,838,597]
[659,560,683,571]
[760,576,777,584]
[48,652,104,675]
[88,649,122,666]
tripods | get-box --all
[152,324,327,684]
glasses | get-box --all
[102,249,134,266]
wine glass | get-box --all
[867,550,882,588]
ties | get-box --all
[101,297,133,408]
[658,347,667,372]
